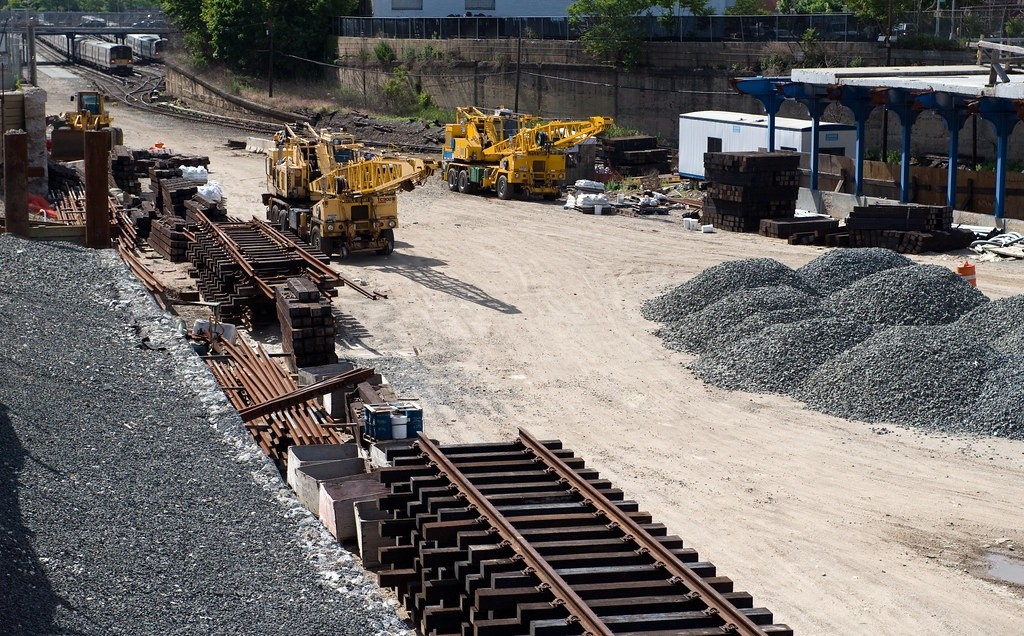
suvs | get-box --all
[824,21,859,42]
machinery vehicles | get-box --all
[262,120,439,259]
[50,90,123,161]
[439,105,613,200]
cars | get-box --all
[148,20,167,28]
[78,19,106,27]
[887,23,919,37]
[728,33,742,42]
[737,26,765,41]
[133,20,152,28]
[764,30,800,41]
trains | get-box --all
[35,34,133,76]
[81,16,169,63]
